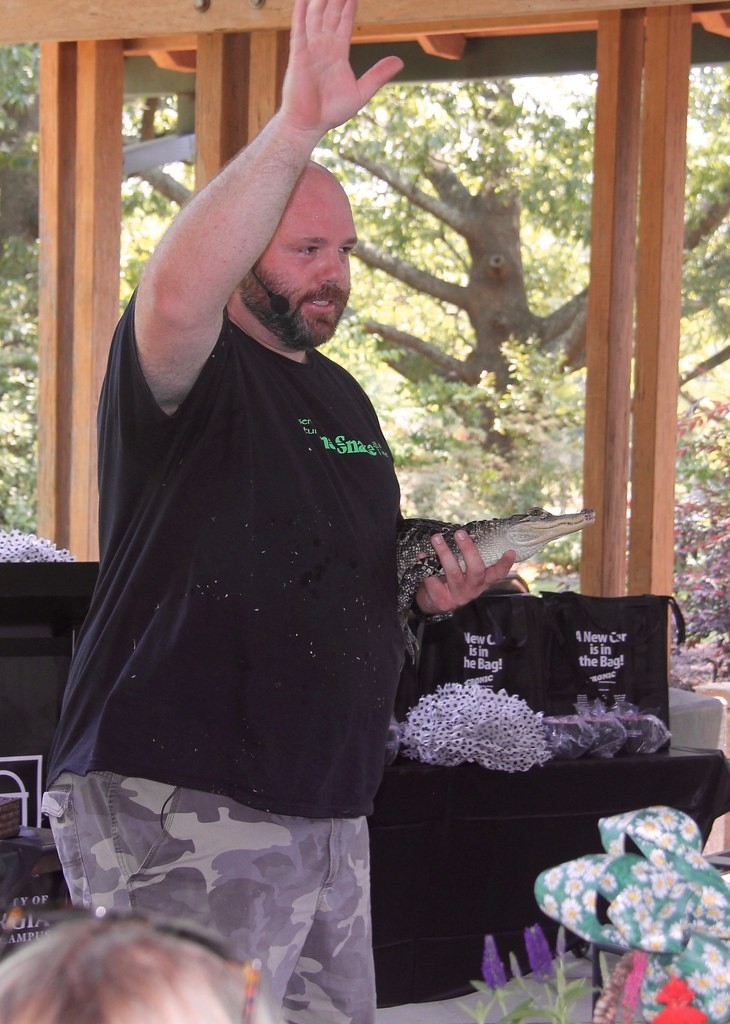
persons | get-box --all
[41,0,518,1023]
[0,916,283,1024]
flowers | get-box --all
[455,923,605,1023]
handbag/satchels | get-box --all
[414,595,544,725]
[538,589,687,750]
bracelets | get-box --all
[411,596,454,623]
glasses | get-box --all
[0,904,262,1024]
